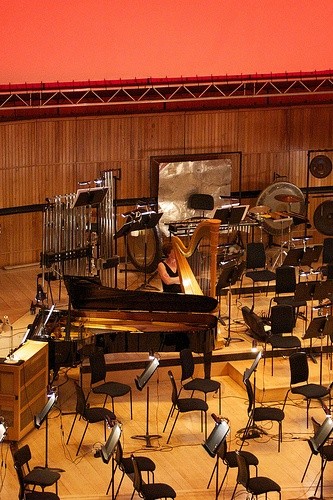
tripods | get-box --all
[130,357,163,446]
[216,257,246,347]
[236,350,268,436]
[293,281,328,363]
[270,218,294,272]
[33,392,66,471]
[131,212,164,291]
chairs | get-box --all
[162,349,222,443]
[186,194,215,222]
[206,241,333,500]
[0,382,176,500]
